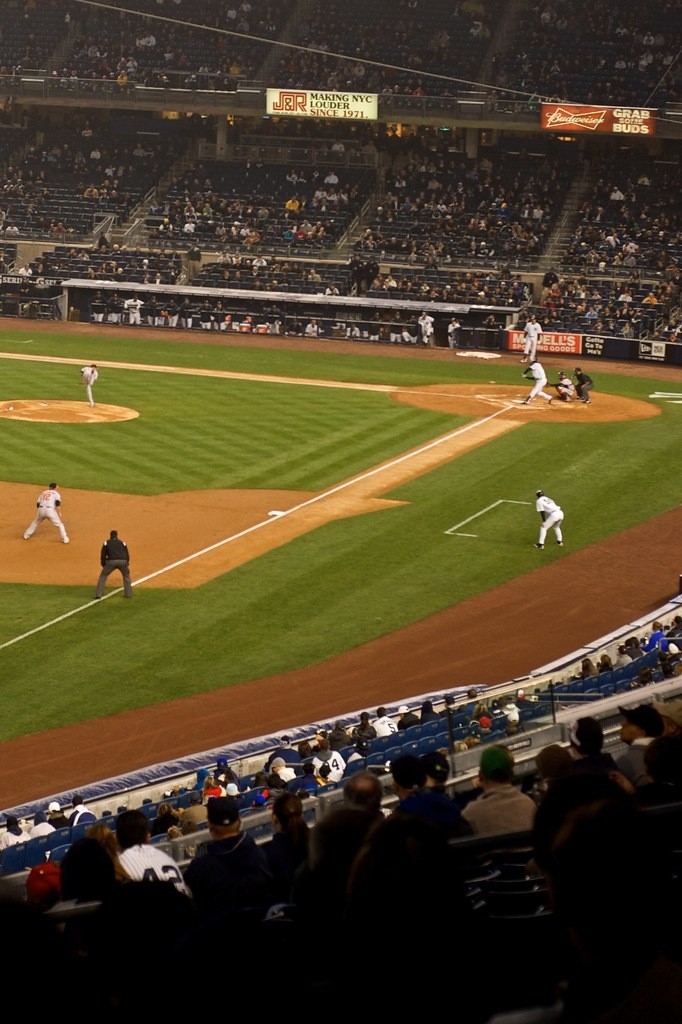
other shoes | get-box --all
[556,541,563,546]
[533,544,544,549]
[577,396,583,399]
[584,399,590,404]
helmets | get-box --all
[558,371,566,380]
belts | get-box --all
[40,505,51,509]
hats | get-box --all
[570,718,604,752]
[421,751,450,782]
[575,368,581,372]
[218,759,227,767]
[480,745,513,781]
[618,704,665,737]
[652,699,682,728]
[537,490,544,496]
[27,861,63,902]
[206,797,239,826]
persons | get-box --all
[94,530,131,599]
[183,697,682,1024]
[0,809,193,1024]
[0,793,99,855]
[519,315,542,364]
[533,489,565,549]
[119,678,519,843]
[0,0,682,346]
[574,616,682,694]
[23,483,69,543]
[522,357,553,405]
[574,368,593,403]
[80,365,99,407]
[550,371,575,401]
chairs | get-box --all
[0,784,681,1023]
[0,0,681,340]
